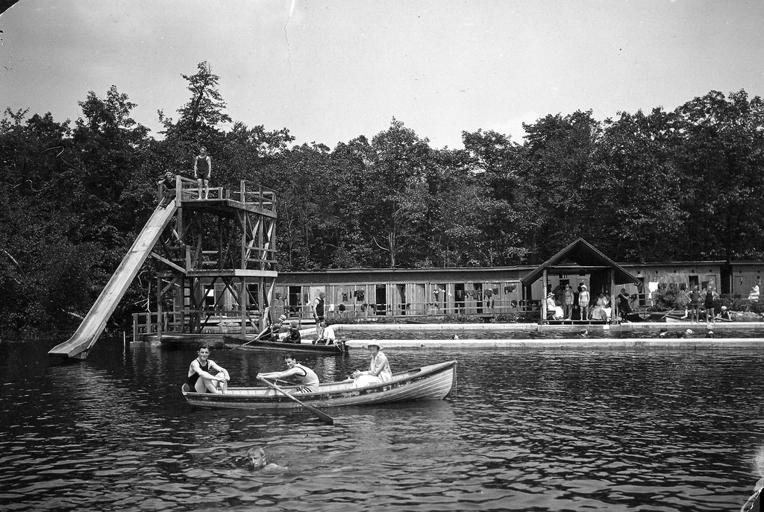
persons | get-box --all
[322,322,335,345]
[745,280,761,312]
[546,284,611,322]
[312,291,326,345]
[256,352,319,393]
[157,172,176,208]
[187,347,230,393]
[349,339,392,388]
[699,285,719,323]
[685,285,704,323]
[615,288,633,323]
[715,306,731,320]
[194,146,211,199]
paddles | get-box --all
[259,377,334,426]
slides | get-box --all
[49,197,177,357]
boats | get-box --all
[160,331,247,350]
[180,360,459,412]
[220,333,351,357]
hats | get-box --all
[581,285,587,288]
[547,292,555,297]
[363,339,384,350]
